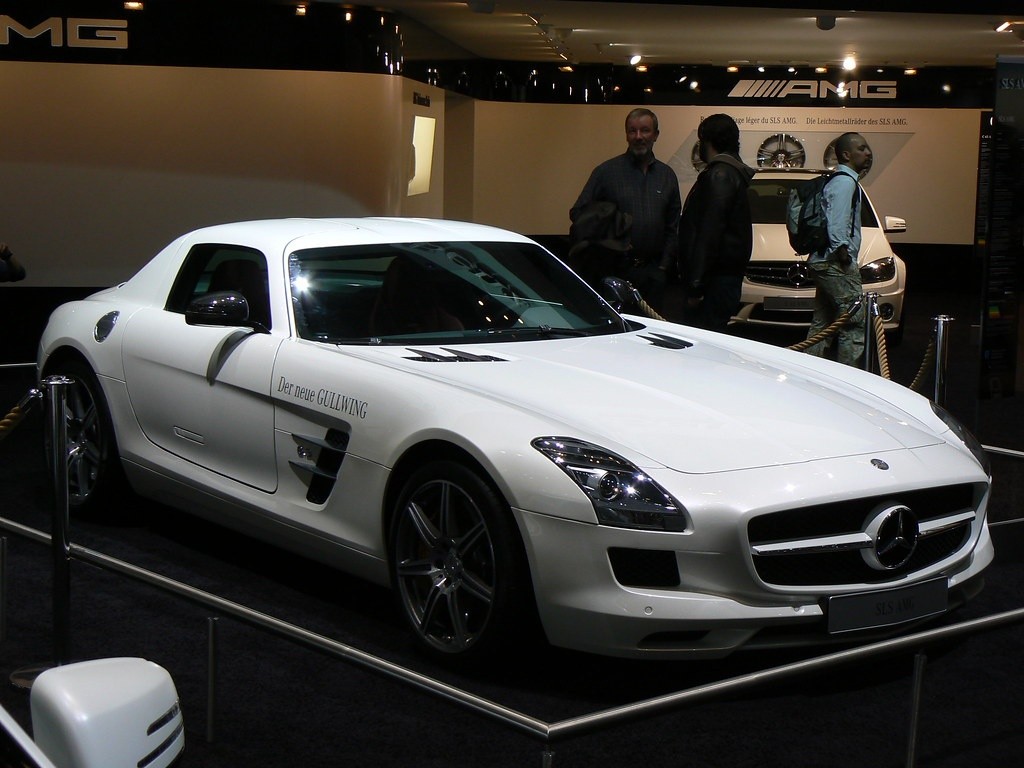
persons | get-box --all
[676,114,755,333]
[570,109,682,319]
[802,132,871,371]
[0,241,25,282]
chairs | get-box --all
[208,259,260,293]
[369,257,464,336]
[301,292,354,338]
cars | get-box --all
[731,167,907,329]
[39,219,993,680]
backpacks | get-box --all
[786,171,860,258]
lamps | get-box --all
[816,16,836,30]
[628,55,641,65]
